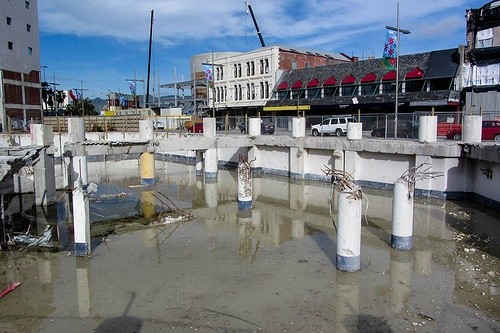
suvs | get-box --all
[311,116,358,137]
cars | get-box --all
[186,119,225,133]
[239,120,249,130]
[482,119,500,143]
[370,121,417,139]
[245,122,275,134]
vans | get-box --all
[412,111,464,141]
